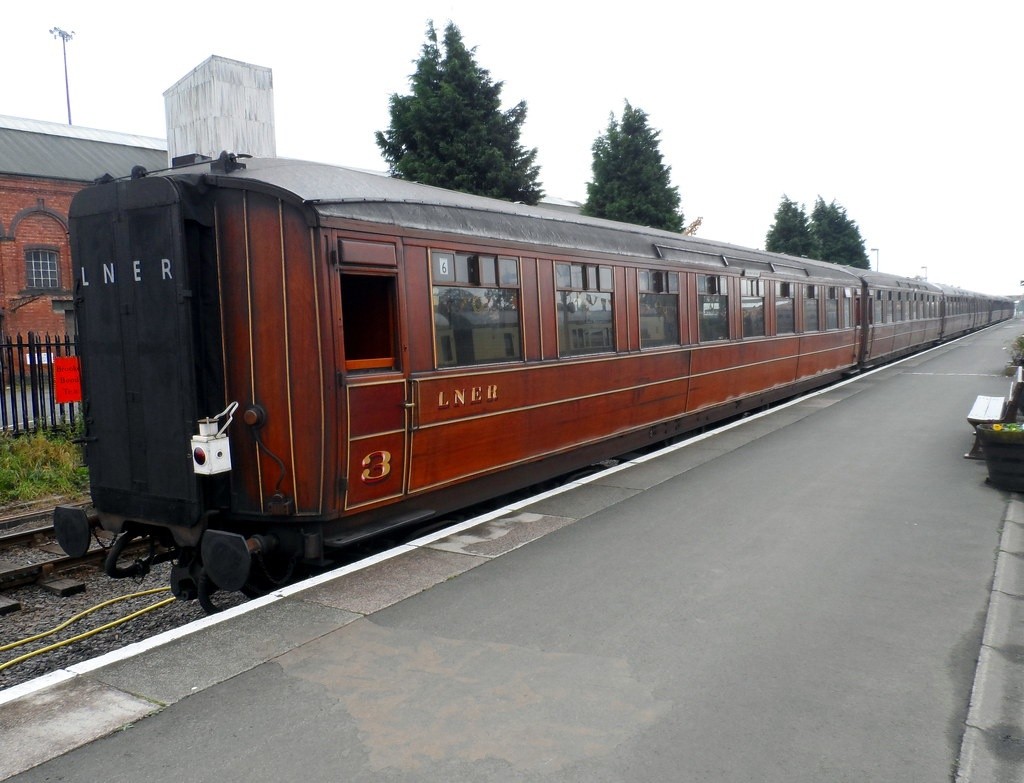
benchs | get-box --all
[963,366,1024,460]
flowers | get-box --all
[992,423,1024,432]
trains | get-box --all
[49,149,1014,615]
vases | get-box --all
[976,424,1024,492]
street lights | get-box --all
[50,26,75,127]
